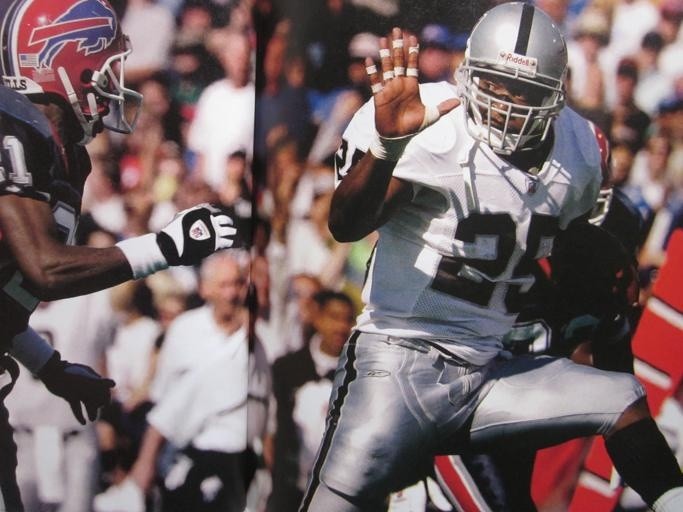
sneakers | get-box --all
[94,477,147,512]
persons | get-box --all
[0,0,683,511]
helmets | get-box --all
[1,0,144,145]
[453,2,567,155]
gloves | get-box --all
[156,203,240,265]
